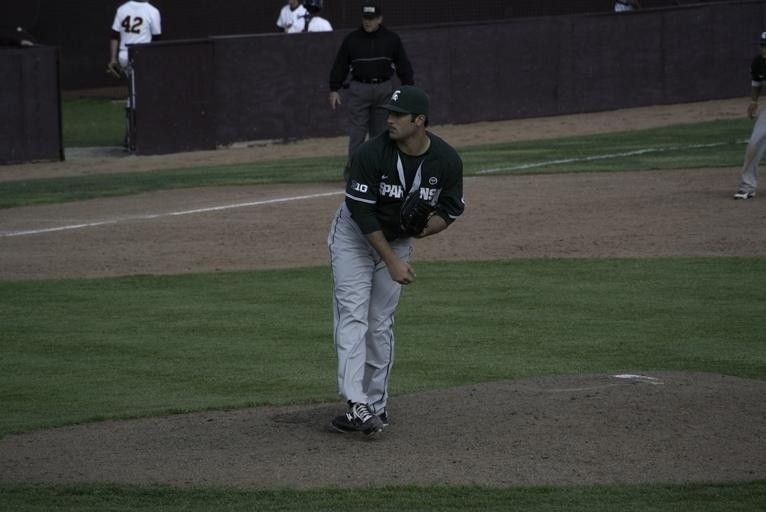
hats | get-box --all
[378,85,430,120]
[361,5,381,19]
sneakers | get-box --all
[733,189,757,198]
[331,401,392,440]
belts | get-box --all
[354,75,388,85]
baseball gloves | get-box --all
[400,189,432,234]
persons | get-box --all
[732,31,766,201]
[104,2,161,153]
[272,1,335,32]
[325,85,464,441]
[327,1,415,186]
